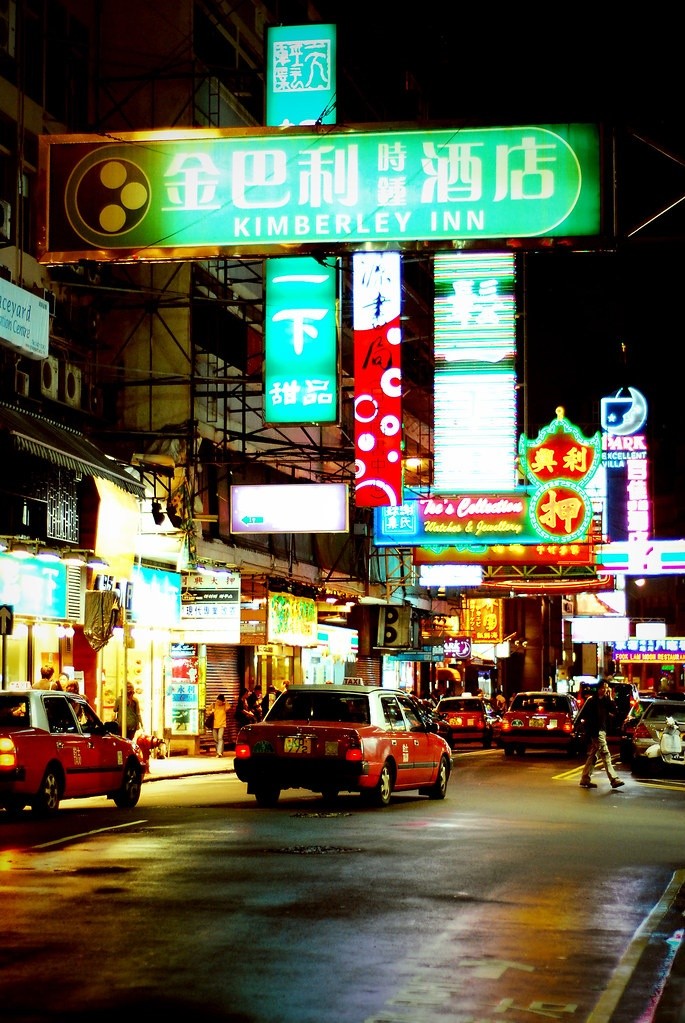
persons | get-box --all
[410,688,454,708]
[490,691,516,717]
[477,690,483,696]
[237,686,282,728]
[32,666,89,724]
[206,694,230,757]
[573,679,624,788]
[114,682,143,740]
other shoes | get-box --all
[611,779,625,788]
[580,782,597,788]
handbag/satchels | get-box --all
[204,703,215,730]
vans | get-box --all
[577,681,638,742]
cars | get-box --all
[433,693,502,749]
[622,692,685,772]
[0,679,147,818]
[501,692,579,758]
[233,678,456,807]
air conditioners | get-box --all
[31,288,57,318]
[40,354,58,400]
[384,606,414,648]
[16,370,29,396]
[59,360,82,408]
